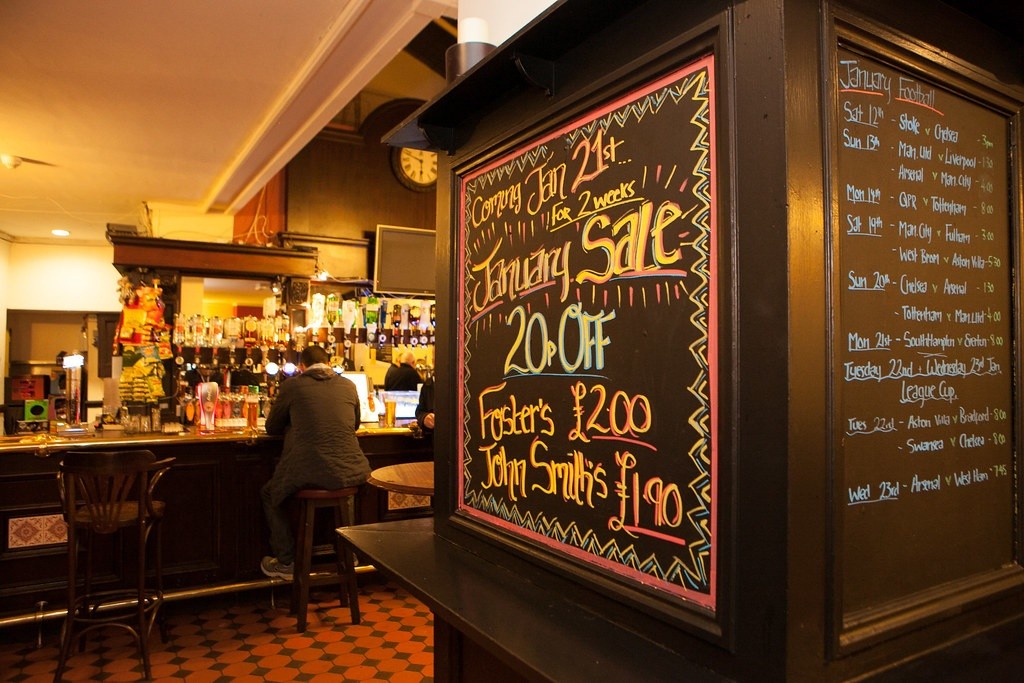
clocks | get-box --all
[389,145,439,193]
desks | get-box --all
[364,462,434,496]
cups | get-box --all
[385,401,395,427]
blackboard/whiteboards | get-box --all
[448,54,733,647]
[825,8,1024,644]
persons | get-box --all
[384,353,403,391]
[415,345,436,461]
[387,352,425,391]
[261,345,372,581]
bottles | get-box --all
[312,294,435,331]
[175,383,283,436]
[173,315,289,346]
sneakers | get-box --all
[261,556,296,581]
[352,552,358,566]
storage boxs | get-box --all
[5,370,68,433]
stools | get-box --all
[291,487,362,632]
[53,450,177,683]
